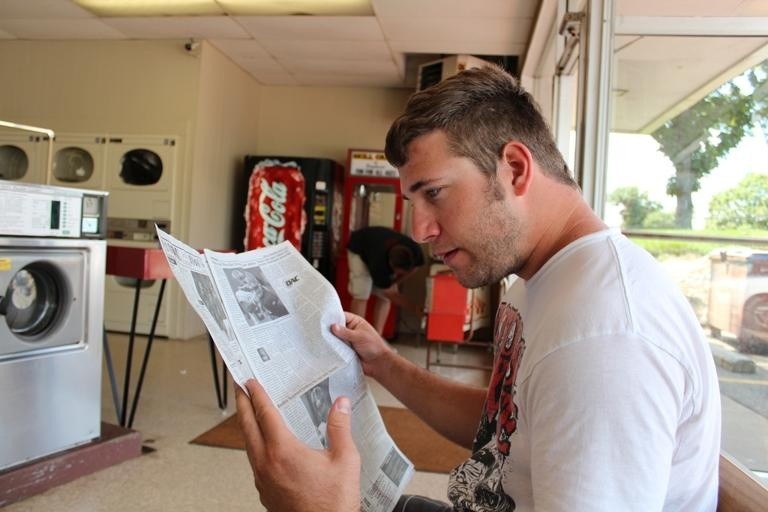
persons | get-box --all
[311,386,330,449]
[232,270,264,314]
[234,64,722,512]
[346,226,425,337]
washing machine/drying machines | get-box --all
[0,181,111,474]
[0,133,182,336]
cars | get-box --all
[667,246,768,351]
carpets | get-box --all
[191,398,479,478]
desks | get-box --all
[99,244,238,434]
